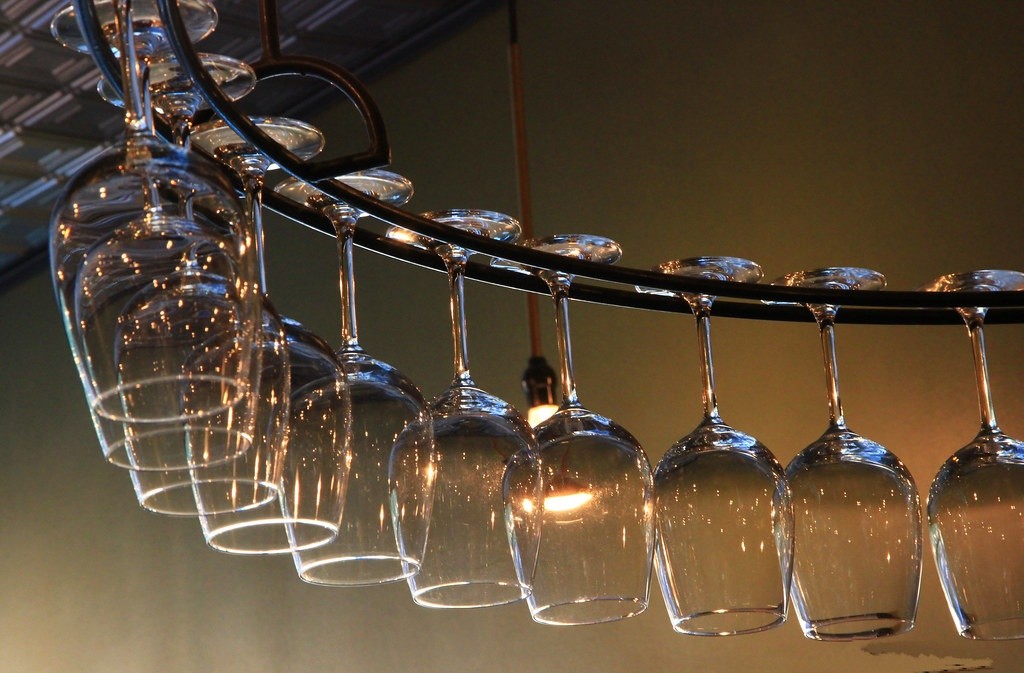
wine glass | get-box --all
[49,0,1024,641]
